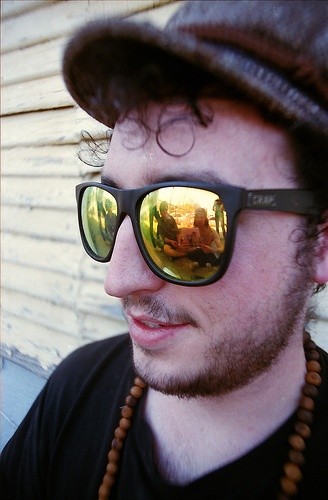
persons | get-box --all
[0,0,328,500]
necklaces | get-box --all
[98,326,321,500]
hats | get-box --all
[61,1,328,136]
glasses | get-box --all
[74,180,324,288]
